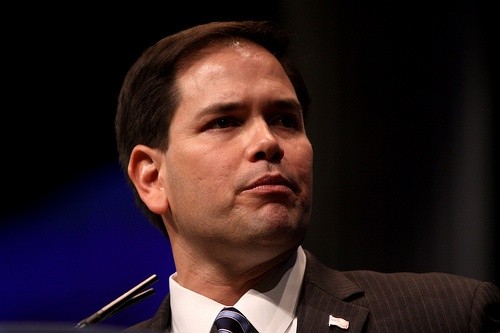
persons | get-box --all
[115,20,500,333]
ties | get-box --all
[210,306,257,332]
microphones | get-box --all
[73,275,158,328]
